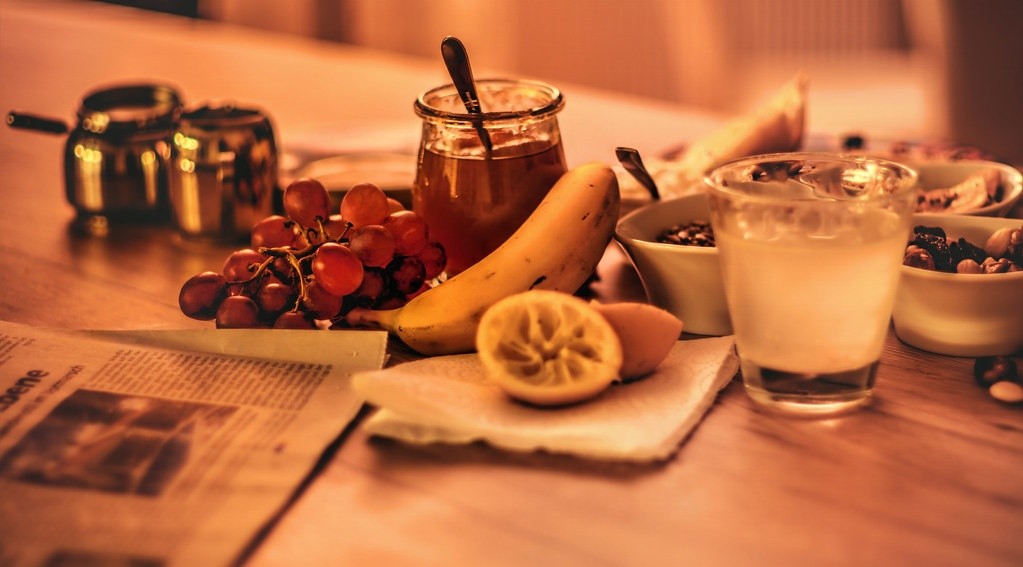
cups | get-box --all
[706,152,918,414]
[415,77,569,288]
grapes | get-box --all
[179,181,446,331]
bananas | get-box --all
[345,165,619,352]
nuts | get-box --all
[903,226,1023,403]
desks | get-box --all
[0,0,1022,567]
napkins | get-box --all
[350,334,738,462]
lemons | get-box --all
[478,287,683,405]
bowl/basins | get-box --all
[891,213,1023,356]
[903,159,1023,218]
[616,191,734,335]
